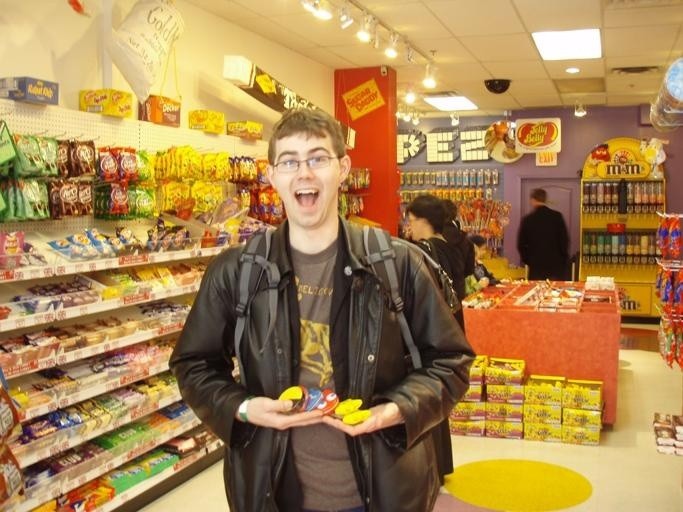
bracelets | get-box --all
[237,394,255,423]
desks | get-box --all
[462,279,620,426]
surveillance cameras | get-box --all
[485,79,510,94]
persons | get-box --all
[169,106,475,510]
[513,187,573,281]
[437,199,478,278]
[401,195,469,487]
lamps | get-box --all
[449,111,460,126]
[302,0,438,88]
[574,99,587,117]
[394,90,423,125]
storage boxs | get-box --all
[0,77,264,139]
[445,355,603,447]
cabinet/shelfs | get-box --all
[578,174,665,320]
[0,226,270,512]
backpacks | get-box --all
[227,225,463,387]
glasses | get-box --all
[274,153,341,173]
